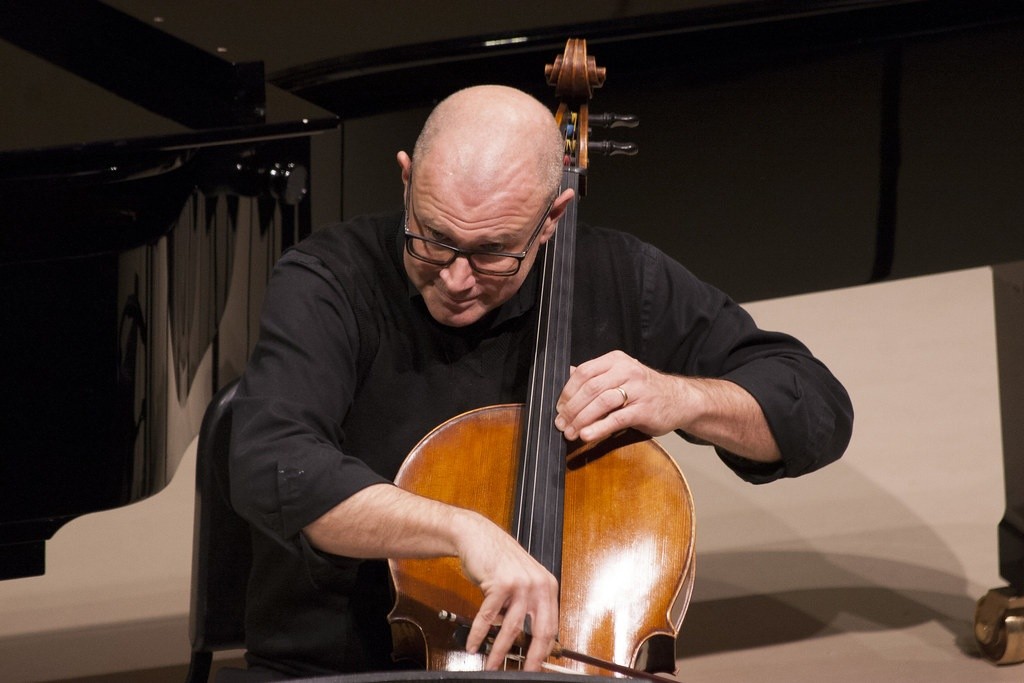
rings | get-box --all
[619,387,628,408]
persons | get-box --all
[229,84,854,683]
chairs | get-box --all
[185,376,247,683]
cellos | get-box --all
[383,37,699,683]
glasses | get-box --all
[401,181,563,279]
